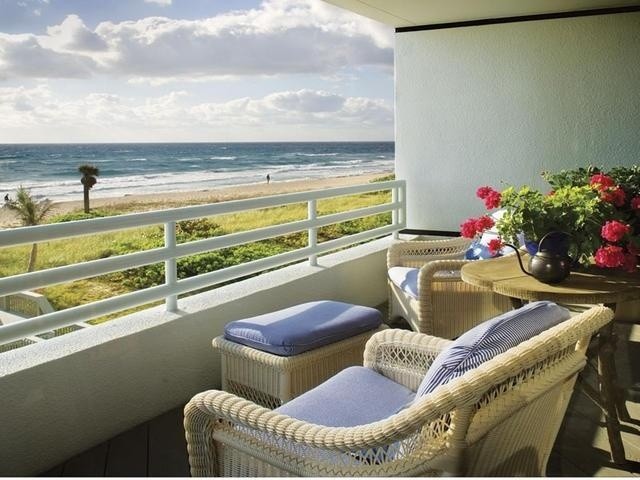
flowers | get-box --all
[455,160,639,272]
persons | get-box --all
[266,174,270,183]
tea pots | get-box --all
[504,234,582,283]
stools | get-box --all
[211,298,391,409]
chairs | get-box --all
[179,293,612,477]
[386,207,527,340]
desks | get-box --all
[457,256,640,477]
